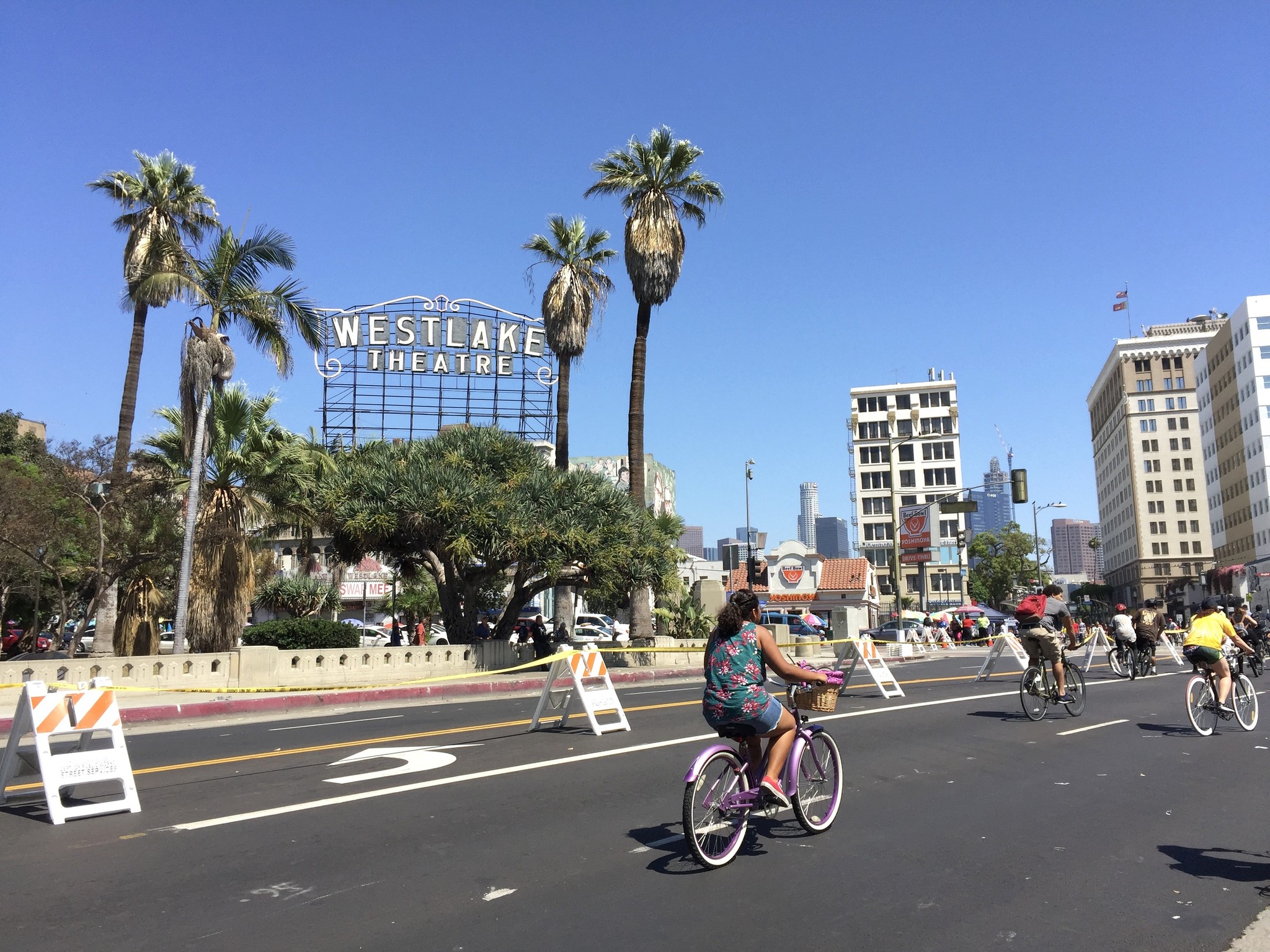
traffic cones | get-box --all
[988,632,994,647]
[941,636,951,649]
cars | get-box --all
[37,626,96,652]
[357,624,407,648]
[859,620,925,644]
[2,629,51,654]
[391,622,451,645]
[157,630,191,654]
[479,612,657,642]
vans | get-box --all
[760,612,826,642]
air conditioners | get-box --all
[908,588,913,592]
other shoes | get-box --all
[1217,701,1235,713]
[1150,671,1158,675]
[1116,649,1123,659]
[1254,654,1262,663]
[1135,666,1139,675]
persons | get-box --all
[923,612,1019,647]
[439,618,443,625]
[525,615,549,672]
[612,617,620,641]
[475,615,491,640]
[1018,584,1076,705]
[1108,598,1179,675]
[390,626,404,646]
[1183,597,1270,714]
[416,617,427,646]
[1061,618,1108,646]
[703,588,829,809]
[553,622,570,643]
[516,622,528,643]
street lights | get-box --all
[887,431,943,629]
[1033,502,1067,592]
[937,568,950,608]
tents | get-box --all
[958,602,1009,632]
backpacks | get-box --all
[923,617,932,627]
[1231,614,1249,638]
[1014,594,1049,623]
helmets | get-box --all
[1144,598,1160,608]
[1114,603,1126,611]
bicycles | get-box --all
[1185,650,1260,736]
[1019,642,1088,722]
[1106,631,1158,680]
[1240,631,1270,677]
[680,653,844,870]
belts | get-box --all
[1020,623,1044,629]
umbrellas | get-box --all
[892,605,982,624]
[795,614,827,626]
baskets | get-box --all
[785,679,840,712]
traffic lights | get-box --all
[956,528,966,549]
[752,556,762,583]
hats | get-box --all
[1169,619,1172,621]
[926,612,930,615]
[1201,597,1218,610]
[1256,604,1263,610]
[980,610,985,614]
[1242,605,1248,610]
[1217,606,1224,610]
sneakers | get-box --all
[1024,678,1037,696]
[1057,693,1076,704]
[759,776,790,808]
